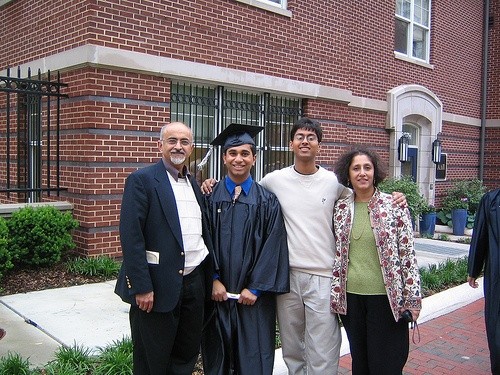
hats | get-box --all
[197,122,265,171]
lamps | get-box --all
[398,136,408,164]
[432,131,442,164]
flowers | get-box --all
[419,199,436,222]
[443,197,469,216]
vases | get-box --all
[451,209,467,236]
[419,212,437,237]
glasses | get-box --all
[293,135,316,142]
[160,139,193,145]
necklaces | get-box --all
[350,212,369,239]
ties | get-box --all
[233,184,243,208]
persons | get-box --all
[332,149,421,374]
[203,123,290,375]
[466,188,500,375]
[200,119,410,375]
[114,122,212,375]
